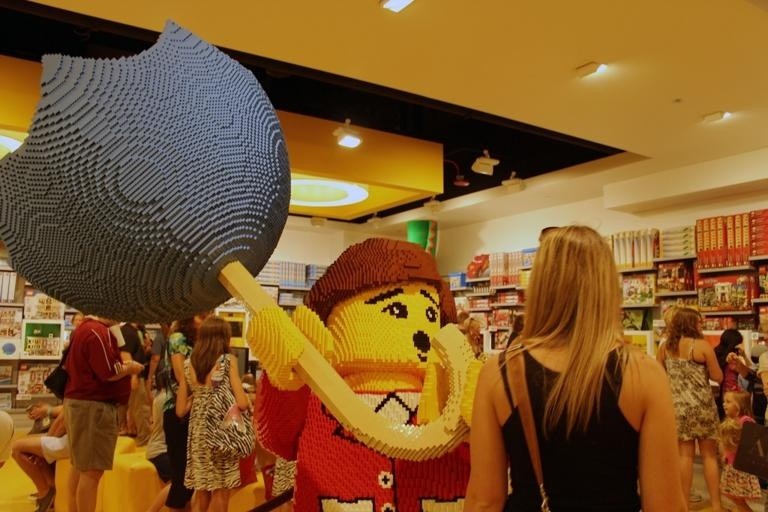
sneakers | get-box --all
[35,485,56,512]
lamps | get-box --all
[470,148,528,196]
[332,118,366,150]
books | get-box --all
[256,260,329,317]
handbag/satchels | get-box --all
[43,344,72,401]
[204,389,258,460]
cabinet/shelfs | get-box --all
[615,251,768,397]
[0,250,83,419]
[448,273,524,355]
[215,283,325,400]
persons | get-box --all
[506,314,524,348]
[655,303,767,512]
[464,223,691,512]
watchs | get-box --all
[457,307,485,362]
